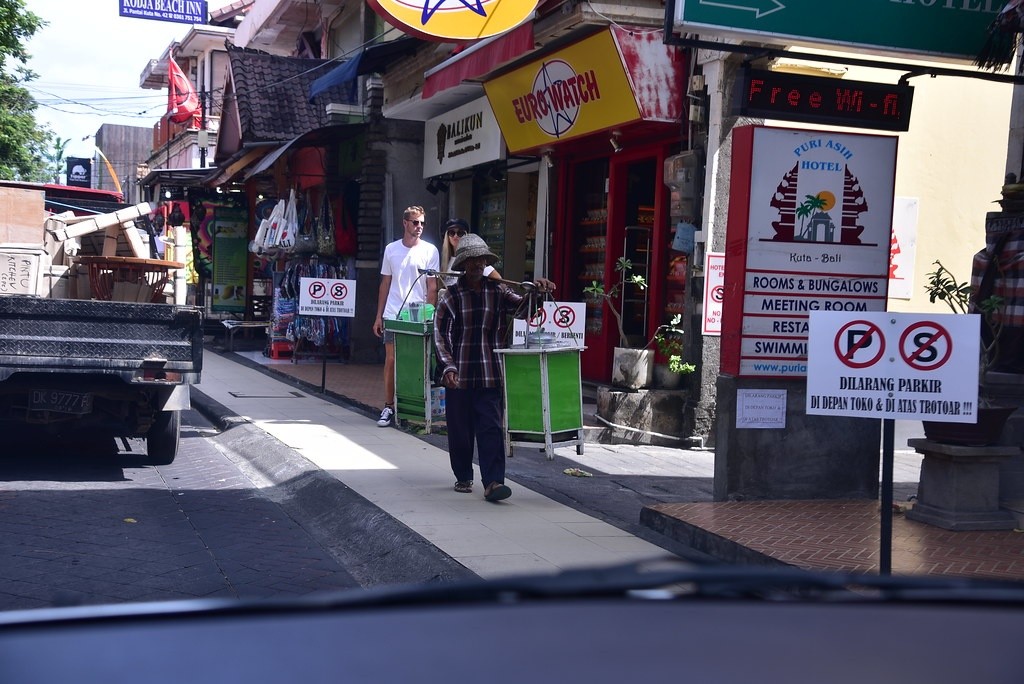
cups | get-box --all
[408,307,419,322]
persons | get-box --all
[440,217,506,281]
[433,233,557,503]
[372,205,441,428]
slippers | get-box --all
[483,481,514,502]
[453,479,474,493]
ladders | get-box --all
[619,226,651,346]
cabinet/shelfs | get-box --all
[475,173,687,368]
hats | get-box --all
[451,233,500,272]
[444,218,469,232]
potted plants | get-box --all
[921,261,1019,448]
[581,256,696,391]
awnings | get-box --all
[422,0,567,100]
[137,123,367,197]
[307,36,425,105]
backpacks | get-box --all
[168,202,185,227]
[279,263,295,300]
[153,212,164,232]
[191,199,206,226]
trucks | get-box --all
[0,291,205,465]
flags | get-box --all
[166,47,206,130]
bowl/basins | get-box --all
[400,308,435,322]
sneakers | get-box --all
[376,405,395,427]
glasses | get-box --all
[447,229,466,237]
[407,220,426,226]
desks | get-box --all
[224,320,270,356]
[73,254,184,301]
